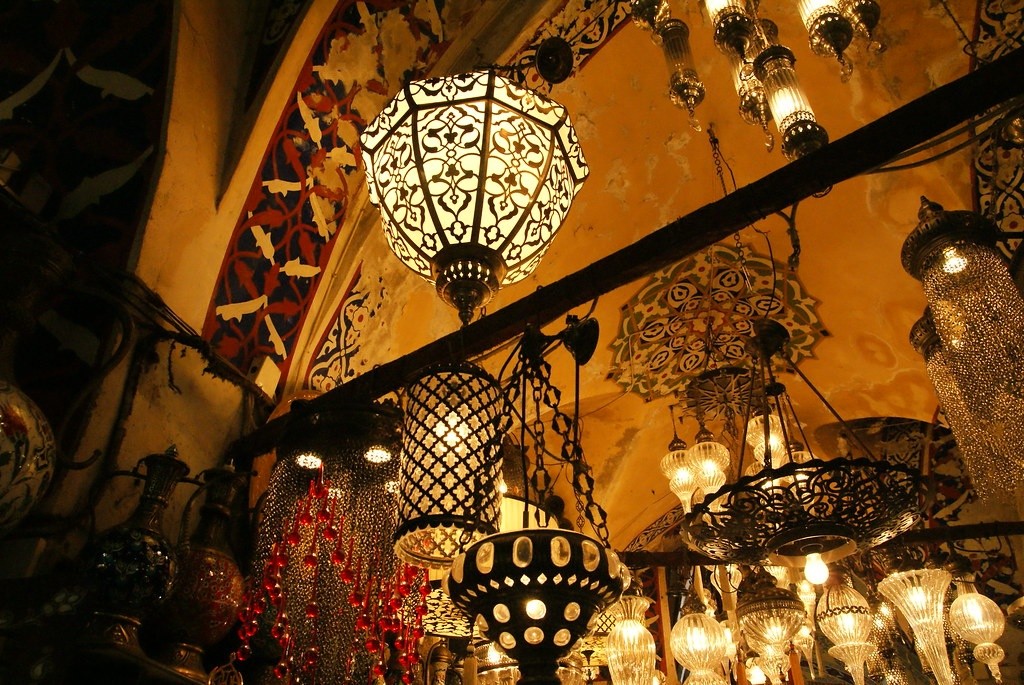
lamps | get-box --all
[237,402,432,685]
[608,196,1024,685]
[359,65,589,329]
[440,324,631,685]
[393,364,504,571]
[633,0,886,163]
